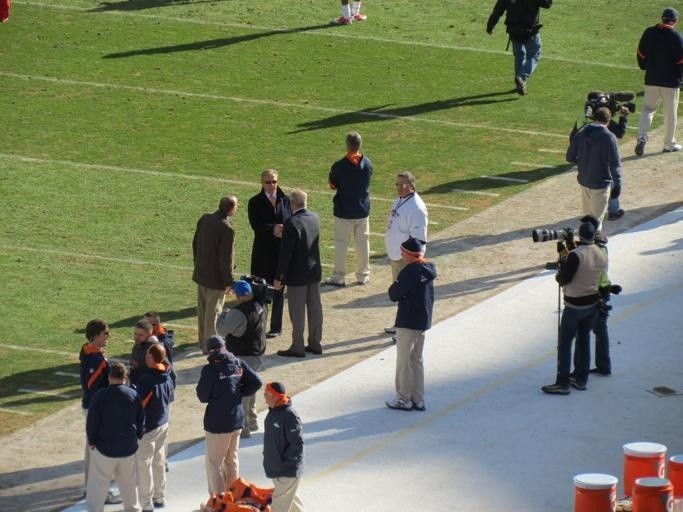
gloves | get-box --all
[610,186,621,199]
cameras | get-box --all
[585,92,636,119]
[240,274,275,306]
[531,226,572,242]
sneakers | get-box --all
[569,372,587,391]
[663,144,682,153]
[589,367,612,376]
[325,277,345,287]
[385,399,413,410]
[392,336,396,342]
[153,497,165,507]
[635,137,646,156]
[411,399,425,411]
[351,14,367,22]
[607,209,624,222]
[105,490,122,505]
[333,16,352,25]
[384,325,396,334]
[542,384,570,395]
[515,74,526,96]
[358,277,370,284]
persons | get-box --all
[262,380,304,512]
[585,91,629,220]
[129,319,176,472]
[86,362,145,512]
[143,310,174,366]
[192,195,238,354]
[634,7,683,157]
[540,223,606,394]
[385,237,437,411]
[486,0,553,95]
[216,280,268,438]
[78,319,122,505]
[566,106,622,232]
[196,334,263,511]
[324,131,373,287]
[274,190,323,358]
[248,169,293,338]
[136,344,174,512]
[384,172,428,342]
[557,214,613,379]
[333,0,367,25]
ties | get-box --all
[269,194,276,207]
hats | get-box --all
[579,215,600,233]
[207,336,225,350]
[231,281,252,295]
[661,9,679,20]
[400,239,424,257]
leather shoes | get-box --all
[265,330,281,339]
[304,344,323,355]
[277,349,306,358]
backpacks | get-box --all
[502,1,543,52]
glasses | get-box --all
[396,183,411,188]
[104,331,109,335]
[263,180,277,184]
